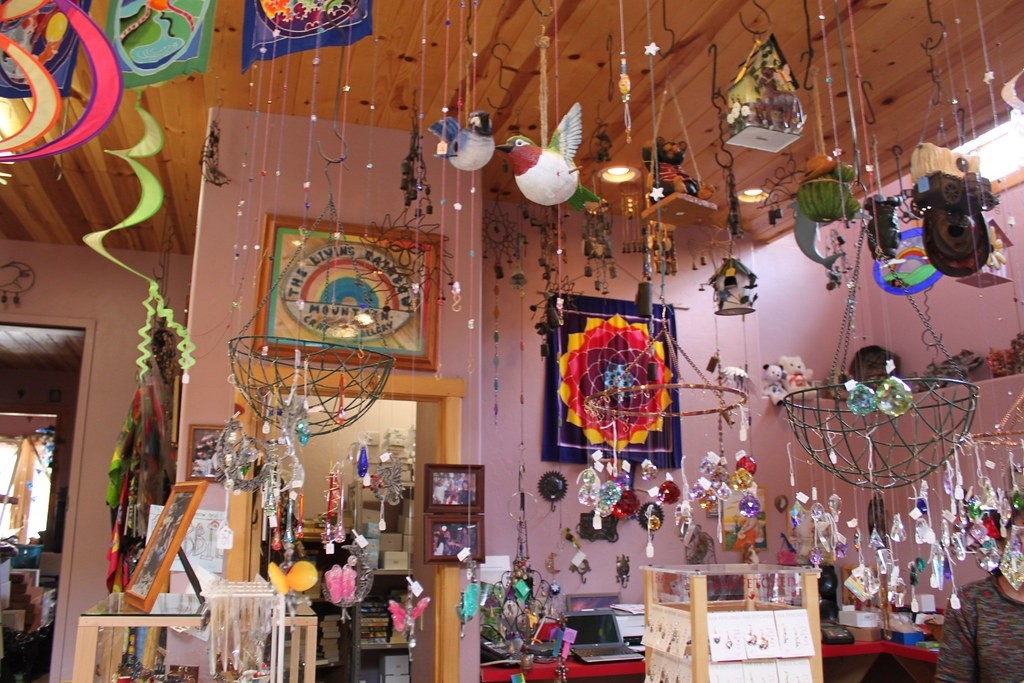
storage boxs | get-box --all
[839,612,924,645]
[0,540,42,635]
[378,652,410,683]
[362,488,415,570]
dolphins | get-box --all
[786,196,844,273]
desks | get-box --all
[480,617,938,683]
[712,610,713,618]
[71,590,318,683]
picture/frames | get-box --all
[252,213,445,374]
[184,423,227,483]
[423,515,486,564]
[122,481,210,613]
[423,463,486,513]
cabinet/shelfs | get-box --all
[263,527,353,683]
[347,481,415,683]
[640,562,826,683]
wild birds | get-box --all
[427,109,495,172]
[494,101,601,213]
